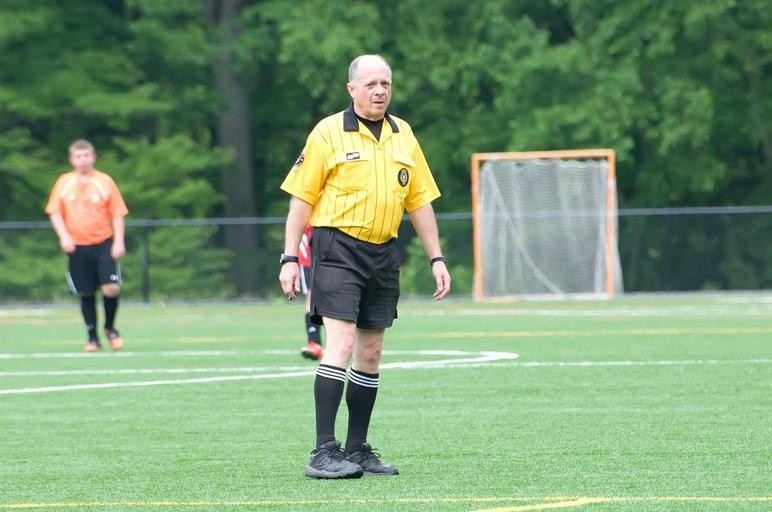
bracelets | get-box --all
[428,256,447,265]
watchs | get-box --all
[280,254,298,265]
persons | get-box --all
[44,139,130,352]
[277,55,451,479]
[289,193,324,359]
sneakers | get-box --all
[82,327,123,352]
[347,441,400,474]
[305,440,363,478]
[301,340,321,358]
